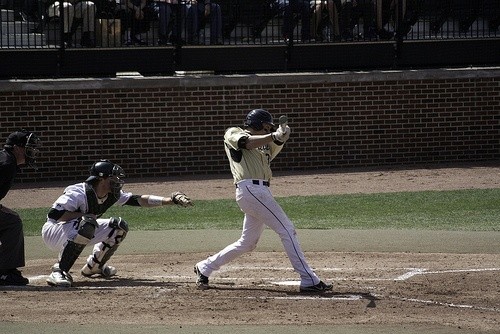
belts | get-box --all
[235,180,269,188]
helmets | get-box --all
[84,160,126,193]
[243,108,276,131]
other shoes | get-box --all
[0,268,30,286]
[193,264,209,289]
[46,263,72,287]
[80,262,118,277]
[298,279,334,292]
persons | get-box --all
[193,109,333,294]
[42,159,193,287]
[0,128,40,286]
[12,0,500,48]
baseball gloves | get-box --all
[171,190,192,207]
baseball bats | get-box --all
[278,115,288,133]
